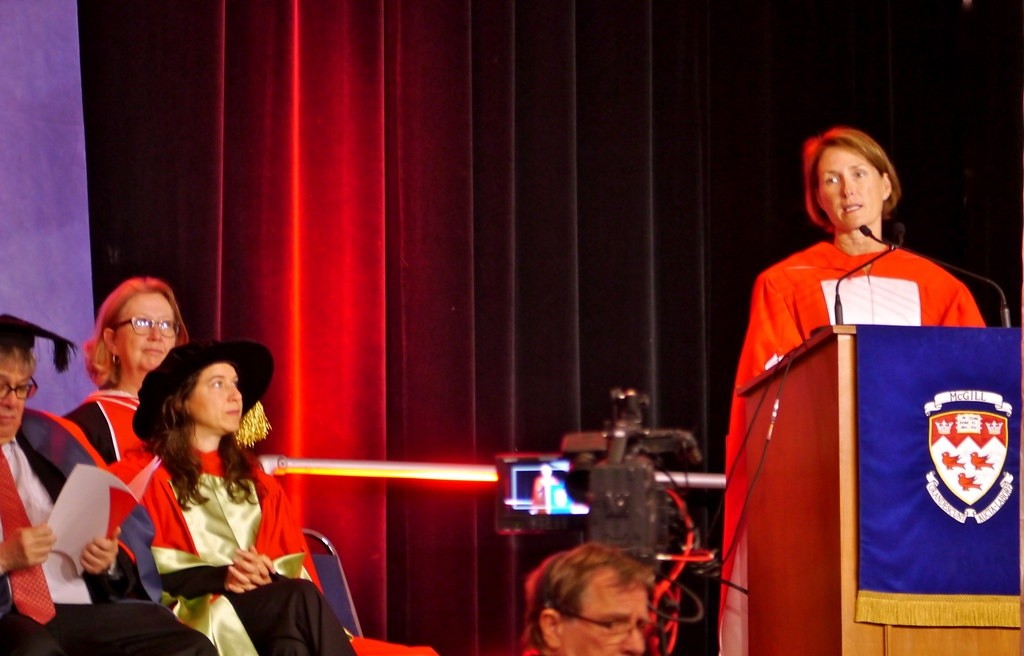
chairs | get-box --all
[301,527,363,638]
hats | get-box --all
[131,340,275,443]
[0,314,78,372]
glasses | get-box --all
[114,315,180,338]
[0,376,38,400]
[562,613,653,634]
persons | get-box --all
[719,127,987,656]
[524,541,655,656]
[530,463,559,514]
[58,277,190,464]
[0,313,218,656]
[105,339,357,656]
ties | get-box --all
[1,446,55,624]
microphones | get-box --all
[834,222,1012,330]
